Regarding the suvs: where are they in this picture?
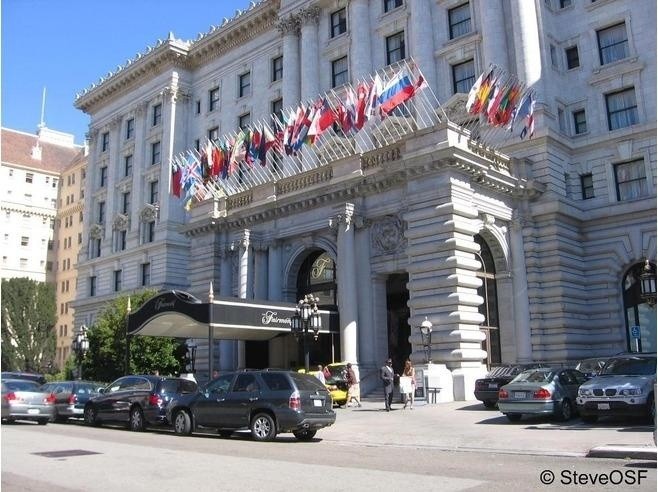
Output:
[164,367,337,443]
[1,371,45,385]
[575,351,656,426]
[40,381,98,424]
[82,374,199,433]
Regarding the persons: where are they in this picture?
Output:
[380,359,400,412]
[402,360,417,410]
[340,363,362,409]
[315,365,327,387]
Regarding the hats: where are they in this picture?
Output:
[384,358,393,364]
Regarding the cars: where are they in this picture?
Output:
[473,362,552,407]
[1,378,55,425]
[96,385,119,394]
[496,366,590,421]
[297,361,358,406]
[572,357,608,375]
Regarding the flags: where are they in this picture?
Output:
[171,59,428,198]
[466,71,537,140]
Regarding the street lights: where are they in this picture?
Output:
[640,260,656,307]
[71,324,91,381]
[288,292,322,373]
[416,316,435,362]
